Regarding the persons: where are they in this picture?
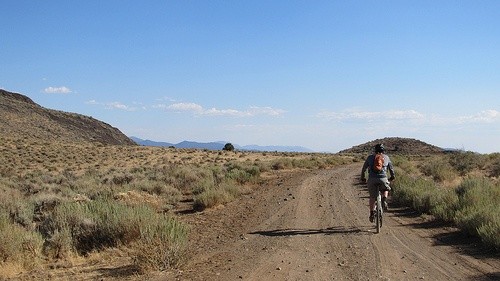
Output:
[359,143,395,224]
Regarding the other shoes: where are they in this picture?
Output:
[382,201,387,210]
[369,210,374,223]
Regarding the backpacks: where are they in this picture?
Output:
[368,154,387,173]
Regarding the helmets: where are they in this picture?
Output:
[375,144,385,151]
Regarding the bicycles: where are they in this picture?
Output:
[362,176,395,233]
